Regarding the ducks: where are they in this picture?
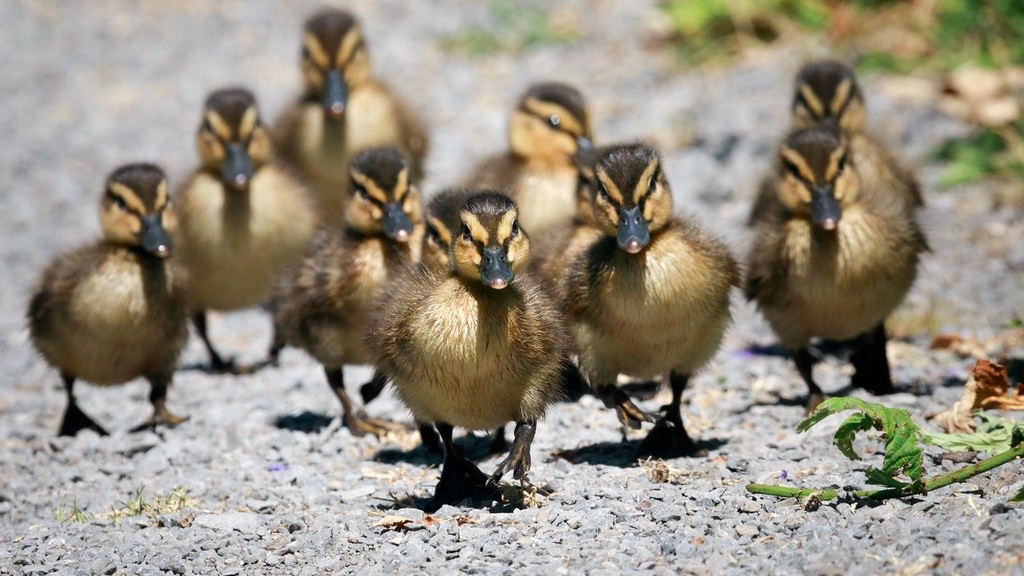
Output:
[25,162,192,434]
[538,139,750,457]
[271,7,429,182]
[273,141,427,437]
[466,79,595,275]
[741,62,931,416]
[364,187,570,511]
[162,88,324,373]
